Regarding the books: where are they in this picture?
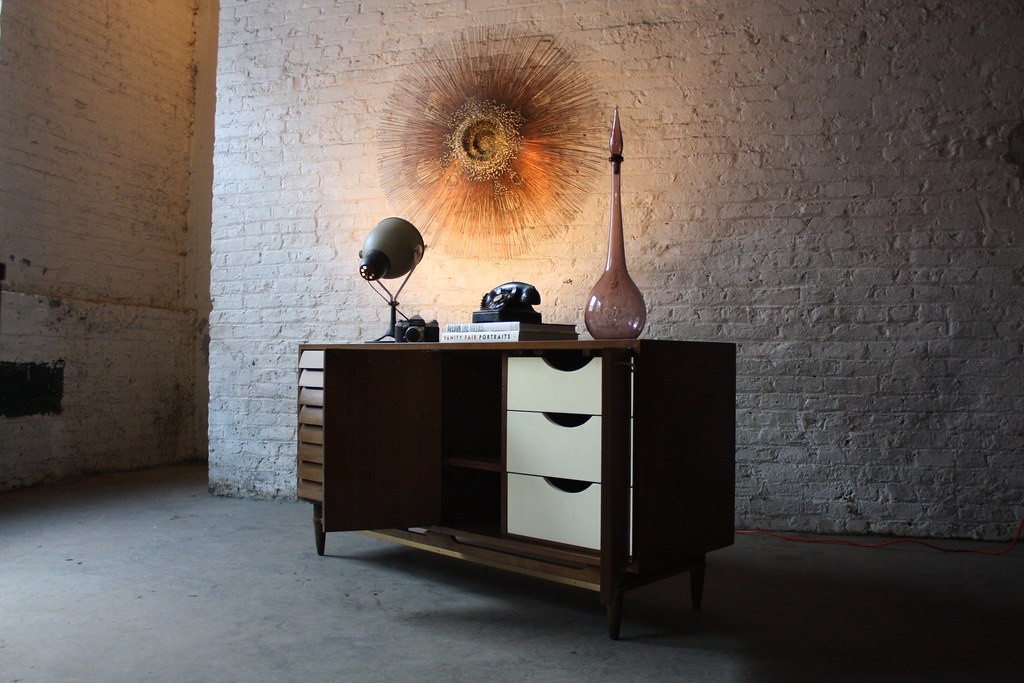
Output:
[438,321,581,343]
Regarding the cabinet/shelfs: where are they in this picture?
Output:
[293,339,738,640]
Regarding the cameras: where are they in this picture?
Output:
[394,314,439,342]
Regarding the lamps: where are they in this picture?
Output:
[359,216,424,343]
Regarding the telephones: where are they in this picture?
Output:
[472,282,542,324]
